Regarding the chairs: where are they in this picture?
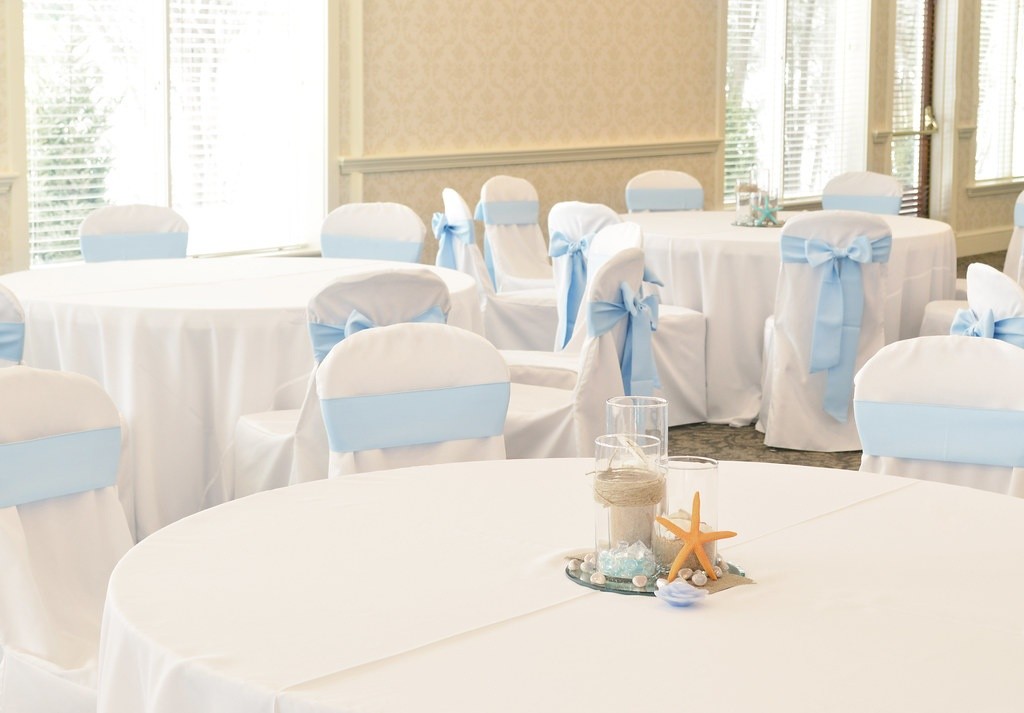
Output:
[0,169,1023,713]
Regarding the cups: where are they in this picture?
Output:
[650,456,718,575]
[750,168,769,217]
[593,433,661,583]
[737,178,761,223]
[768,187,778,218]
[606,396,669,518]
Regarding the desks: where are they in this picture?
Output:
[0,255,476,543]
[98,457,1022,713]
[619,208,957,429]
